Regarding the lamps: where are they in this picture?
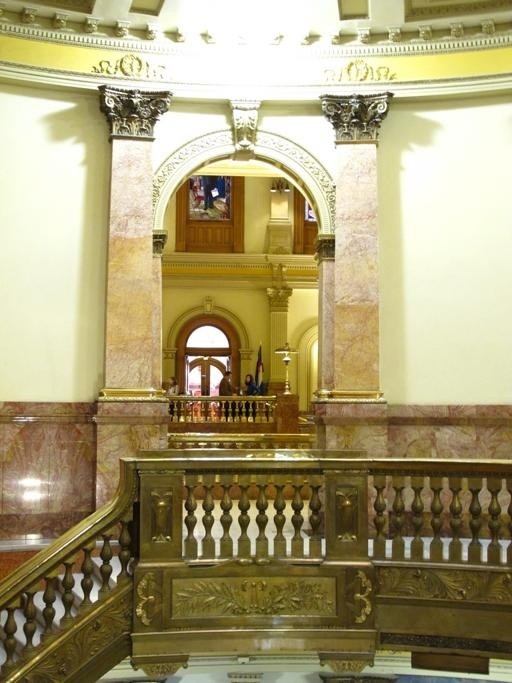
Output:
[269,177,290,194]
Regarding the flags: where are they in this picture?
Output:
[254,344,265,394]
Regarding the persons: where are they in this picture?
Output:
[190,175,230,213]
[244,373,261,395]
[166,376,181,396]
[219,370,239,396]
